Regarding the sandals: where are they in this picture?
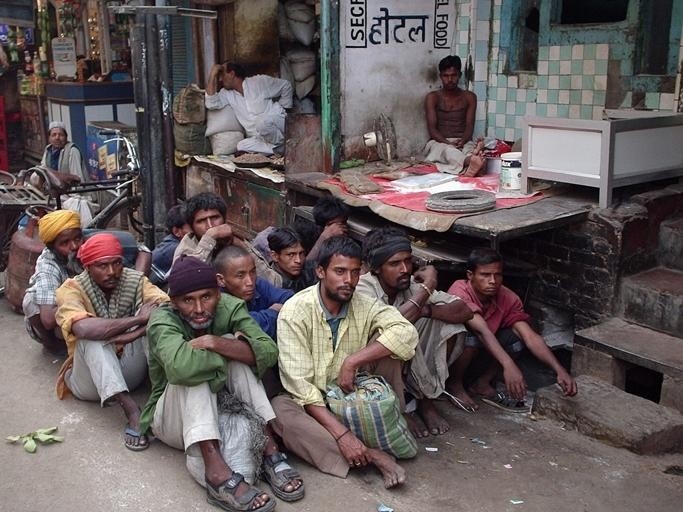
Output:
[259,452,304,502]
[204,470,276,512]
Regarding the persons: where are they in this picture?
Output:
[22,192,579,512]
[422,55,486,178]
[205,60,293,157]
[28,121,97,203]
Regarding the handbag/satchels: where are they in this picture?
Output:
[327,371,418,460]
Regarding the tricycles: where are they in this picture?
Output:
[1,128,144,275]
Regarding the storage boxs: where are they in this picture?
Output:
[87,121,135,197]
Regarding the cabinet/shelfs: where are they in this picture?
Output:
[18,96,49,168]
[45,81,135,179]
[184,160,287,242]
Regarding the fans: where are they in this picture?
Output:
[363,113,397,163]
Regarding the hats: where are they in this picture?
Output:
[49,120,67,131]
[168,254,217,298]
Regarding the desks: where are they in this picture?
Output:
[521,116,683,209]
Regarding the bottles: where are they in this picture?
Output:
[0,2,80,98]
[106,14,130,72]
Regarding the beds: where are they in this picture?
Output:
[318,165,570,251]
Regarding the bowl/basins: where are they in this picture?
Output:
[232,158,284,171]
[485,158,502,174]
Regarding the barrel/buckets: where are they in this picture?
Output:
[500,151,522,191]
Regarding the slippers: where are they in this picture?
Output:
[481,392,530,413]
[124,422,149,451]
